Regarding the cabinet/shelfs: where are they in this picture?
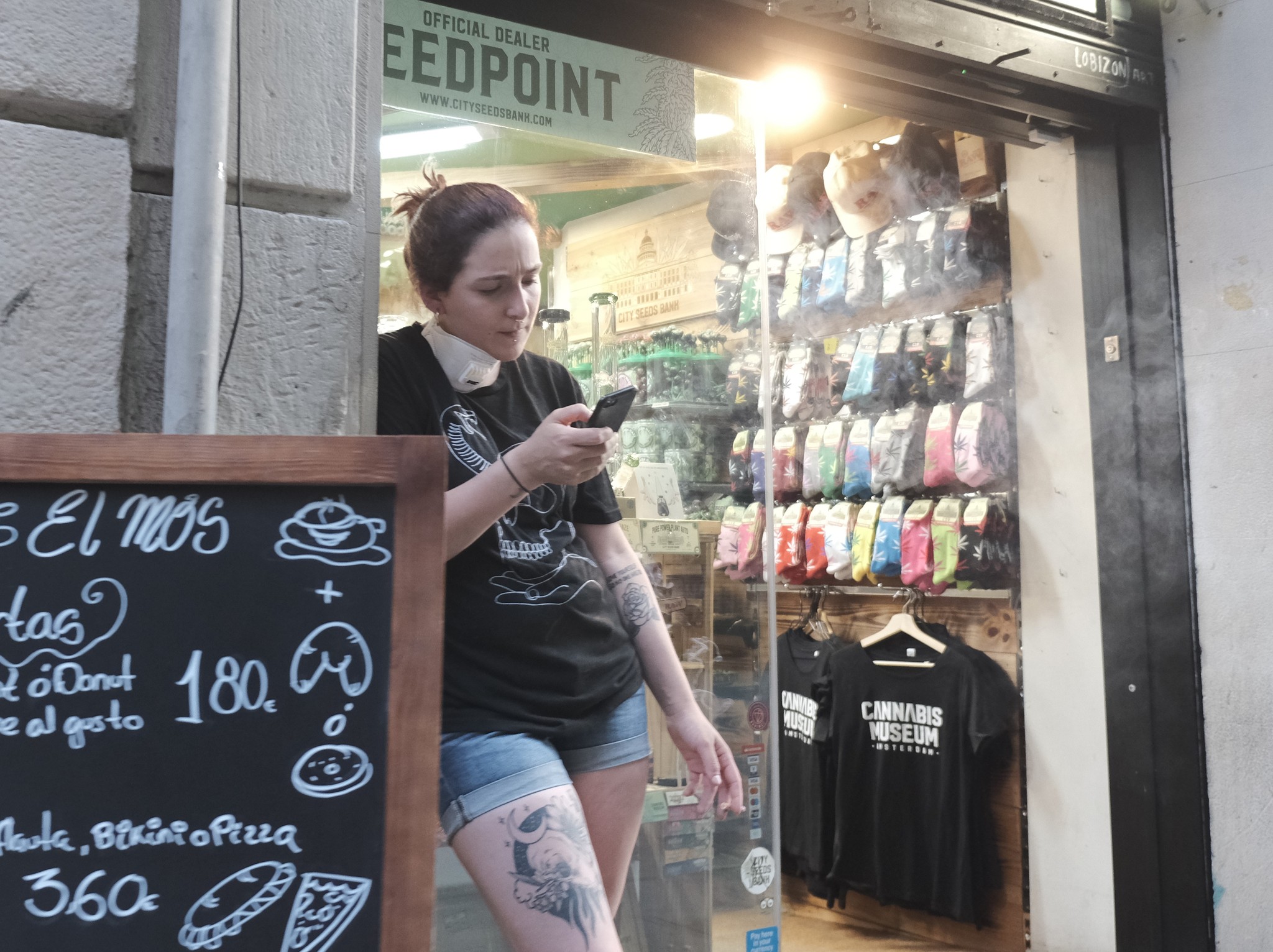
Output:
[565,347,734,515]
[620,519,770,904]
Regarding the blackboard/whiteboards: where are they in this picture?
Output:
[0,433,448,952]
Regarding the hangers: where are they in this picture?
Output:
[788,587,948,668]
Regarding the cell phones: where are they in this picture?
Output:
[585,385,637,432]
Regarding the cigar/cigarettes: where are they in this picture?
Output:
[721,804,744,813]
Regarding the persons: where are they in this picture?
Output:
[376,167,745,952]
